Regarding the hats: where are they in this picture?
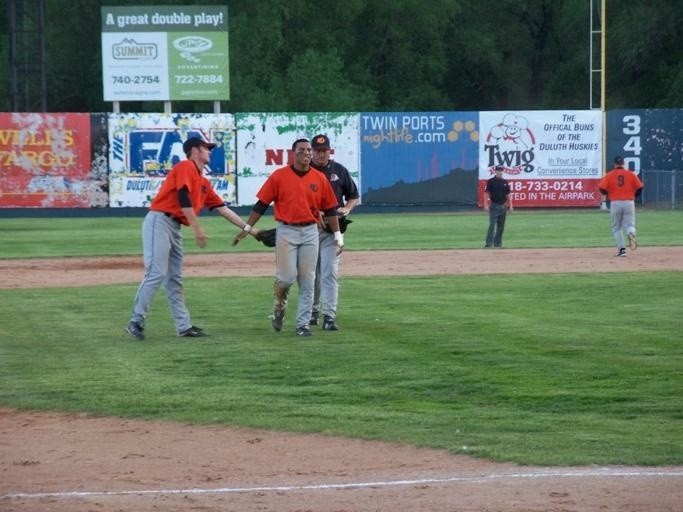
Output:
[183,137,217,153]
[311,135,330,151]
[613,156,624,165]
[495,165,504,170]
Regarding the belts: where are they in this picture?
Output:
[278,219,315,227]
[164,212,182,224]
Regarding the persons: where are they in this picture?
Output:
[232,138,344,336]
[598,155,644,257]
[482,165,513,249]
[307,133,361,331]
[123,138,275,343]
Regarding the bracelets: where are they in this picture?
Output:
[243,223,251,232]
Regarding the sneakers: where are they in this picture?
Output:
[295,324,314,337]
[177,325,212,337]
[323,316,340,332]
[124,321,144,341]
[616,249,627,257]
[485,244,501,248]
[309,312,320,326]
[628,232,637,251]
[272,310,284,332]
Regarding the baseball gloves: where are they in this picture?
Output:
[256,228,276,247]
